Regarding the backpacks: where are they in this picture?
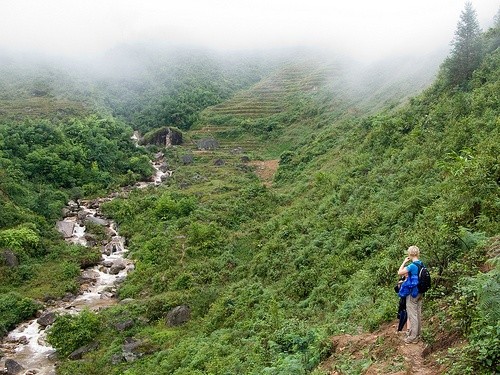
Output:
[408,262,431,293]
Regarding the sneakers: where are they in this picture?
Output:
[404,337,417,344]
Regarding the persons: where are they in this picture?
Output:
[394,265,410,332]
[395,245,428,345]
